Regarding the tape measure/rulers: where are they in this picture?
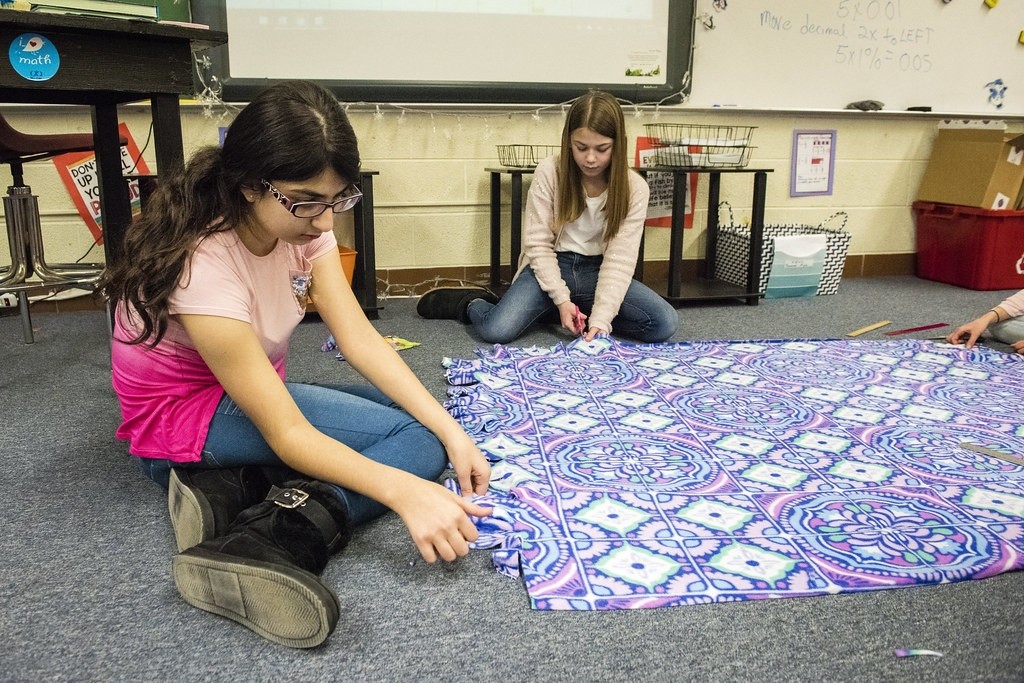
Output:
[960,442,1024,467]
[845,320,892,337]
[883,323,950,335]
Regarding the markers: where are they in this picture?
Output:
[712,104,737,107]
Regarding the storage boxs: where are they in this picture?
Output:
[916,119,1024,210]
[912,201,1024,291]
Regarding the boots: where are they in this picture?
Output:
[172,481,350,648]
[168,465,304,553]
[417,287,499,324]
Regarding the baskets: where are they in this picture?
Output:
[714,201,852,295]
[646,121,759,170]
[497,145,562,168]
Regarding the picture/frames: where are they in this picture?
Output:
[789,129,837,197]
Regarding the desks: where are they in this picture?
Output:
[123,168,383,319]
[484,164,775,311]
[0,11,229,334]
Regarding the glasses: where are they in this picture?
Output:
[258,178,363,218]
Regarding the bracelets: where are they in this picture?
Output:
[990,309,1000,324]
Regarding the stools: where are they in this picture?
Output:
[0,105,128,345]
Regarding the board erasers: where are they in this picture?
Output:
[907,107,931,111]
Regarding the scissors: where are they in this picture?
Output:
[575,306,584,340]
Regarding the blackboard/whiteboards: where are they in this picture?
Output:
[682,0,1024,122]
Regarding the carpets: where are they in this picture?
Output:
[439,335,1024,616]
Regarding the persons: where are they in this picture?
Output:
[945,289,1024,354]
[416,90,679,345]
[90,79,493,649]
